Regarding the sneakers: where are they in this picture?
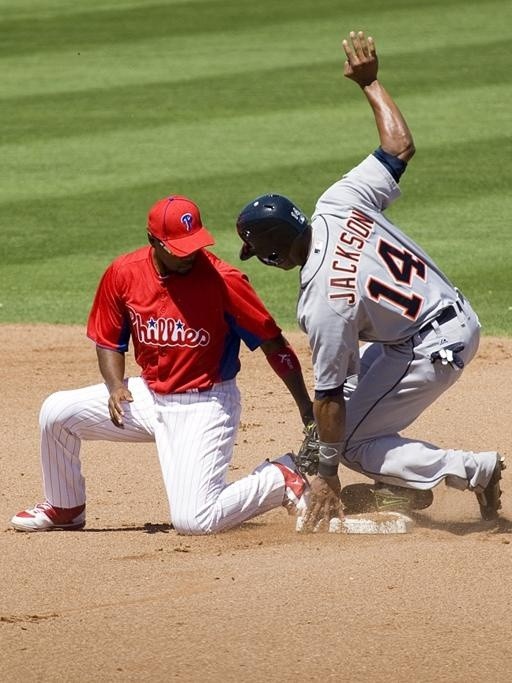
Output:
[341,484,433,513]
[11,502,86,532]
[474,452,507,520]
[270,453,313,532]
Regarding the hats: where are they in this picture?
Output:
[148,195,214,258]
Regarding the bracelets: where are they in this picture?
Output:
[303,406,315,426]
[313,437,345,479]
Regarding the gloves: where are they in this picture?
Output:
[431,345,464,371]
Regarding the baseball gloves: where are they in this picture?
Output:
[294,408,319,477]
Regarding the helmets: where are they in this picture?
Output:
[236,195,309,266]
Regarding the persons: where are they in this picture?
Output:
[10,194,316,535]
[234,29,506,535]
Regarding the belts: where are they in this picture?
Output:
[419,288,463,333]
[175,384,213,393]
